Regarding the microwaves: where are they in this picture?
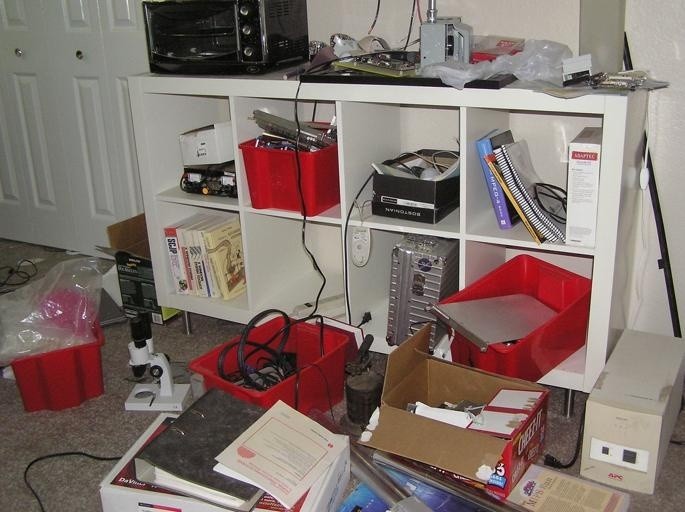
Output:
[142,1,310,76]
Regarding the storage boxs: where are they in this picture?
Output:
[236,118,340,216]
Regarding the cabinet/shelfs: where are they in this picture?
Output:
[129,70,647,417]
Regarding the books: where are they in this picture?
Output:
[475,129,566,246]
[133,387,631,512]
[163,212,247,301]
